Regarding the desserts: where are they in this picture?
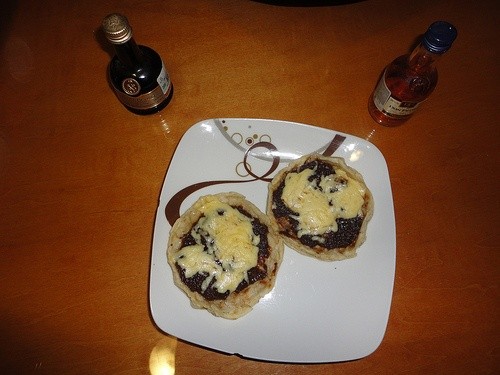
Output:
[166,191,285,320]
[267,152,374,261]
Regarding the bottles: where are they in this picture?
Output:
[103,12,174,117]
[367,20,460,128]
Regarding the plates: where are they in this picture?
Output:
[149,117,398,363]
